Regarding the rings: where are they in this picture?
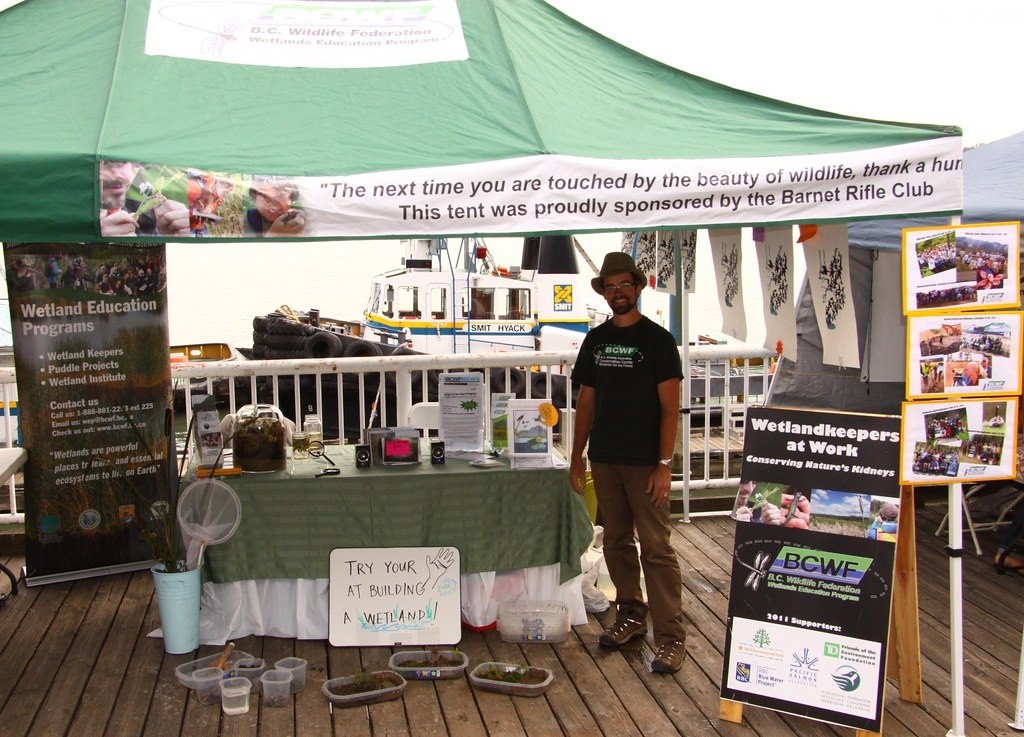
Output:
[664,494,668,497]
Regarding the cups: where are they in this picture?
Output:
[219,676,252,716]
[274,656,308,693]
[191,666,224,706]
[260,669,294,706]
[233,657,266,693]
[151,559,202,654]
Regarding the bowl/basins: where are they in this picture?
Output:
[175,649,254,689]
[497,599,572,644]
[470,661,554,697]
[388,650,469,681]
[320,669,407,709]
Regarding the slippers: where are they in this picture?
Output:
[992,557,1024,576]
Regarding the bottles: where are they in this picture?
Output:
[292,432,310,459]
[304,414,323,450]
[232,404,288,474]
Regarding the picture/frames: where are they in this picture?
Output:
[906,311,1024,398]
[901,221,1020,316]
[899,397,1019,486]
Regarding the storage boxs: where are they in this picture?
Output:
[586,542,648,603]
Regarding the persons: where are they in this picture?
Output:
[781,493,810,529]
[917,242,956,274]
[867,502,898,541]
[188,171,235,236]
[6,250,166,295]
[569,251,687,673]
[99,159,191,237]
[916,285,977,305]
[242,176,308,236]
[913,414,1004,476]
[959,248,1006,290]
[919,333,1003,388]
[736,479,780,525]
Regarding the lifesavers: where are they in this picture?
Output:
[386,347,421,385]
[303,332,342,358]
[494,367,526,394]
[264,316,313,334]
[492,265,523,279]
[264,346,314,392]
[531,372,559,398]
[429,369,439,385]
[344,338,385,384]
[262,333,308,350]
[251,314,268,359]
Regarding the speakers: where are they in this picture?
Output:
[431,442,445,464]
[356,445,371,468]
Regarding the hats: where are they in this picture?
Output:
[591,252,647,295]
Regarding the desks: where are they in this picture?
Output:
[182,445,595,645]
[0,448,28,595]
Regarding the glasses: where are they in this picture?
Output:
[603,281,636,293]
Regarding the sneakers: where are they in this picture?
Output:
[650,638,686,673]
[598,609,648,646]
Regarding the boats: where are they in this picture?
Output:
[243,234,601,413]
[169,342,240,411]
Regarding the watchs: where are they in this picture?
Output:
[658,459,674,469]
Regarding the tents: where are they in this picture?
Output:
[764,130,1024,415]
[0,0,963,737]
